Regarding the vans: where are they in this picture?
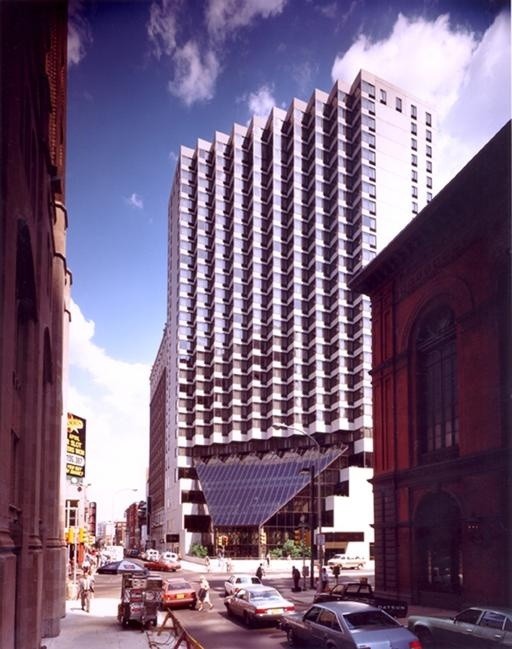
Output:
[327,554,366,570]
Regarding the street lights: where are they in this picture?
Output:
[273,423,322,591]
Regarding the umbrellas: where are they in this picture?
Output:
[98,559,149,574]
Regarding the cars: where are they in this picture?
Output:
[224,574,512,649]
[159,577,197,611]
[124,549,181,572]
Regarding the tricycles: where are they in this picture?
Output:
[118,573,162,632]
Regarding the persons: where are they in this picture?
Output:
[256,563,266,579]
[205,556,211,573]
[266,553,270,564]
[197,576,214,610]
[226,557,233,571]
[291,566,301,586]
[322,567,328,589]
[76,569,96,613]
[82,559,90,571]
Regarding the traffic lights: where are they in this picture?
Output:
[67,526,87,545]
